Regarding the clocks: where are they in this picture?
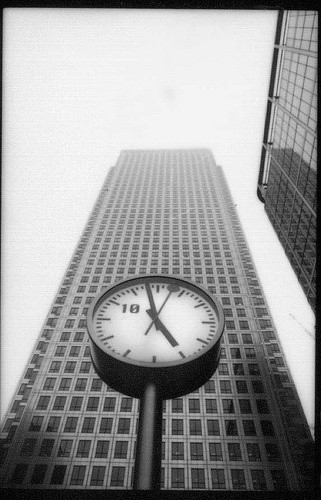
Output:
[86,272,227,369]
